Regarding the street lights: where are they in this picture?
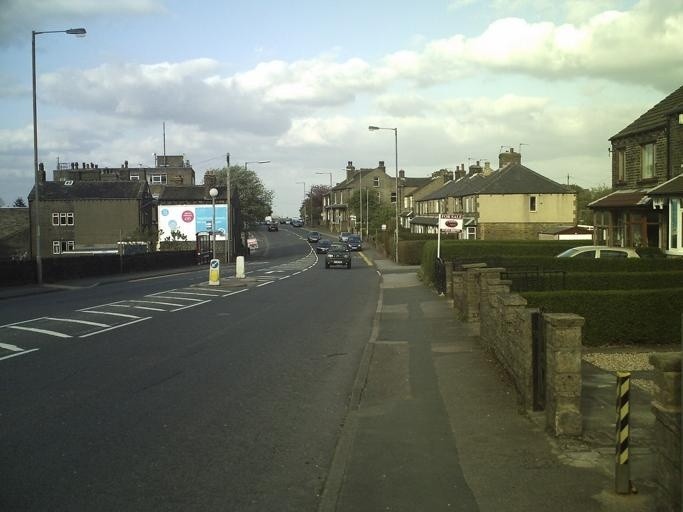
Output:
[368,125,399,266]
[294,181,305,216]
[315,172,332,231]
[245,160,271,170]
[209,188,218,259]
[32,28,87,284]
[340,168,362,231]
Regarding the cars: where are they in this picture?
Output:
[306,231,361,269]
[264,216,304,232]
[537,225,607,241]
[555,246,640,258]
